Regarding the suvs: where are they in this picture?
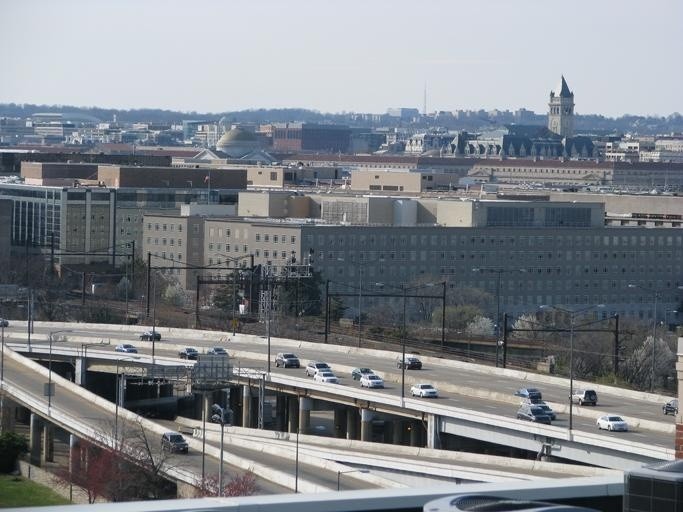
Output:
[568,390,597,406]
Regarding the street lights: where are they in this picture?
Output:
[115,356,141,437]
[628,283,682,393]
[49,329,73,405]
[202,388,230,496]
[238,269,297,372]
[296,426,325,494]
[1,304,24,381]
[471,267,526,367]
[539,303,605,432]
[337,257,387,348]
[337,469,369,491]
[375,281,434,399]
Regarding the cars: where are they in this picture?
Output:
[139,331,160,341]
[114,344,136,353]
[0,318,8,327]
[178,347,198,359]
[160,433,188,453]
[275,353,438,399]
[662,400,677,416]
[596,416,628,432]
[207,347,227,356]
[517,387,555,425]
